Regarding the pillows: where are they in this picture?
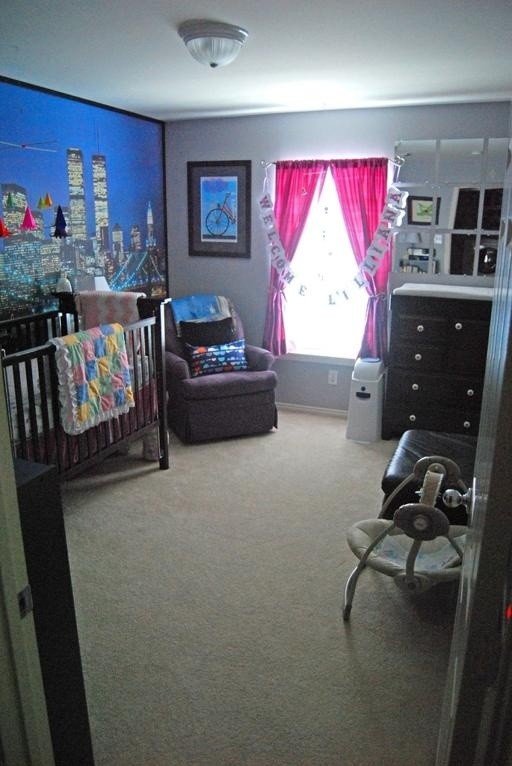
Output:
[185,337,249,378]
[179,316,236,349]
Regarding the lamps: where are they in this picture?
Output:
[180,23,249,71]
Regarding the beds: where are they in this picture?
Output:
[0,291,172,484]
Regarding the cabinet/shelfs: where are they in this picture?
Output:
[381,294,493,441]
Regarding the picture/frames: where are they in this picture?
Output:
[186,159,252,258]
[407,195,441,226]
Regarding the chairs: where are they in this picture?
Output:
[158,295,279,445]
[375,428,477,524]
